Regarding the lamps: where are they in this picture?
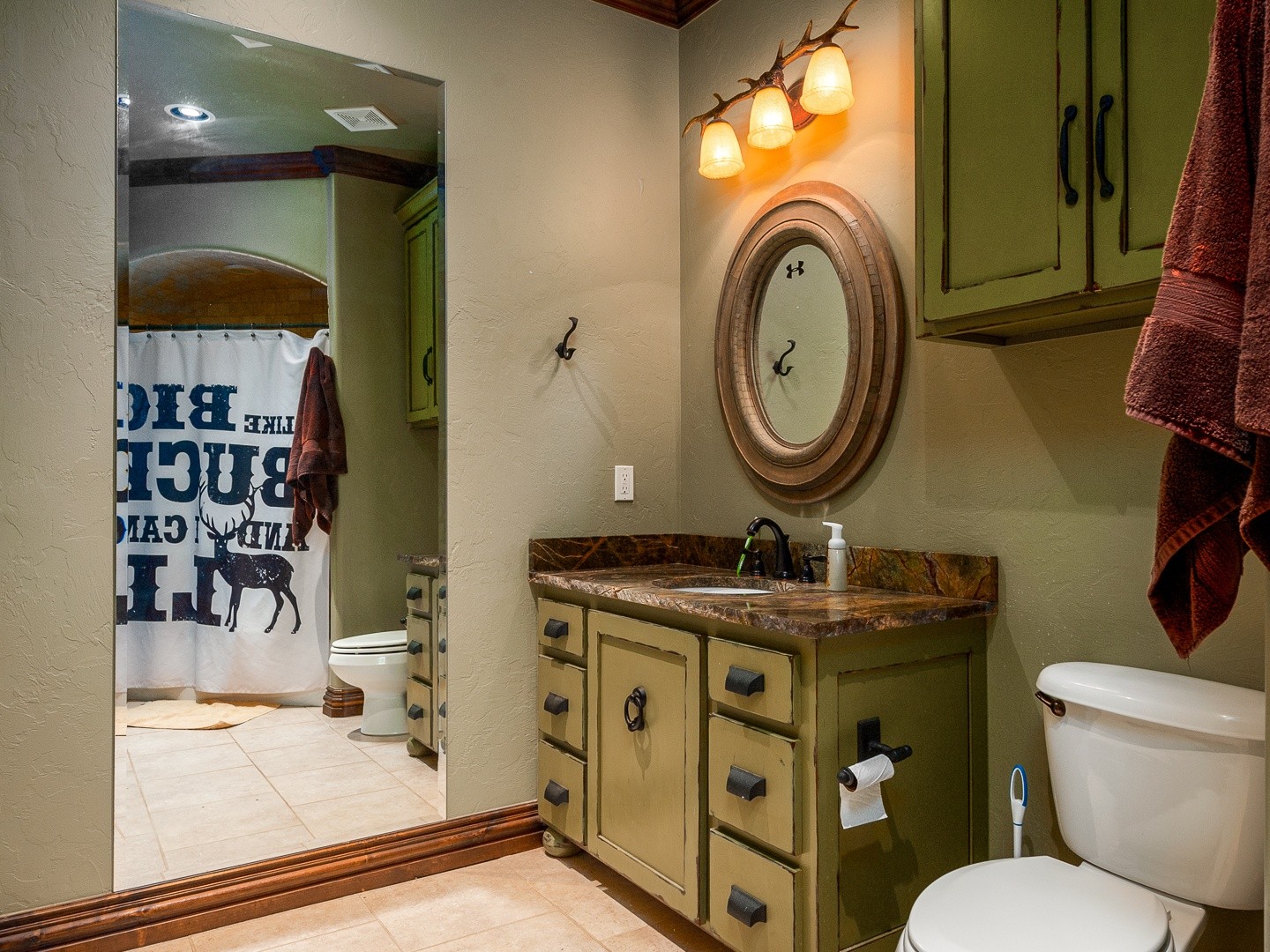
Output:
[698,117,745,179]
[747,83,797,150]
[800,41,854,115]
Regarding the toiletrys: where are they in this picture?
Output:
[821,520,852,593]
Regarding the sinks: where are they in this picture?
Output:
[652,574,798,597]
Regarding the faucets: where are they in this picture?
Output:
[747,517,797,580]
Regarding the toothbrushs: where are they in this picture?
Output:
[736,515,762,576]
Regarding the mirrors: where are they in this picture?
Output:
[713,179,906,503]
[116,0,449,892]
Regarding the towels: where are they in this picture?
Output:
[1119,2,1269,659]
[285,346,349,549]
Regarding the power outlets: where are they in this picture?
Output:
[615,466,634,500]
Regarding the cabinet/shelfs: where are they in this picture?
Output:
[529,531,998,951]
[914,0,1216,351]
[396,554,445,758]
[393,176,440,429]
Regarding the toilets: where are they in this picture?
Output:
[889,661,1270,952]
[327,623,420,739]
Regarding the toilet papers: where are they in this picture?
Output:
[836,754,897,831]
